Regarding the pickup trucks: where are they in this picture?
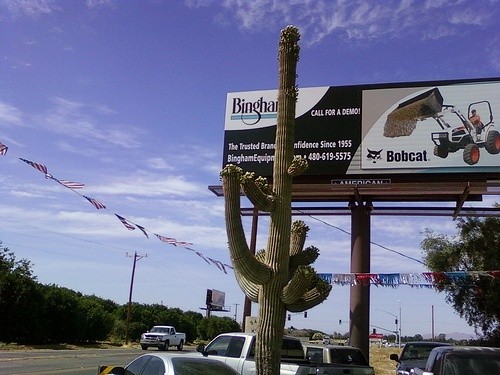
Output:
[139,325,186,350]
[185,332,376,375]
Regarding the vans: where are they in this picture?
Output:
[302,344,369,366]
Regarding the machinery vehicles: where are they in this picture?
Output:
[386,86,500,166]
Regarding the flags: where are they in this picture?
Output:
[0,142,8,155]
[83,195,107,209]
[19,157,53,179]
[137,224,194,246]
[58,180,85,189]
[186,247,228,275]
[115,213,135,230]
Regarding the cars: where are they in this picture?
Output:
[323,337,330,344]
[407,345,500,375]
[390,341,452,375]
[97,352,241,375]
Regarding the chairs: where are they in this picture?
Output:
[226,337,241,356]
[410,350,418,358]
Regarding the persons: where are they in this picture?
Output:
[469,109,480,124]
